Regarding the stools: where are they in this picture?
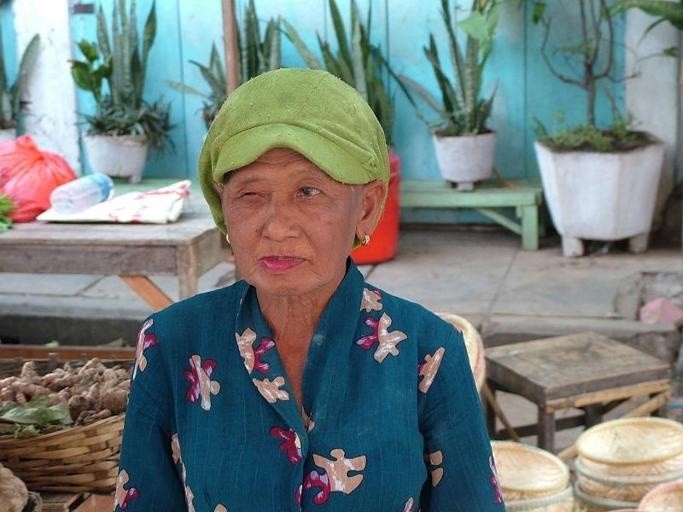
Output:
[398,179,546,248]
[478,329,673,479]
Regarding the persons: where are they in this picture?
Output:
[112,67,506,512]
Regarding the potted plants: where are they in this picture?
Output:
[532,0,679,260]
[396,0,508,193]
[253,0,396,258]
[2,0,40,143]
[66,0,181,189]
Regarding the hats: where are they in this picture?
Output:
[197,67,392,254]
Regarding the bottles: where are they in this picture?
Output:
[48,173,114,214]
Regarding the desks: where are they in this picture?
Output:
[0,201,232,362]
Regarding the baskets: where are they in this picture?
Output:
[488,416,683,512]
[429,309,488,397]
[0,352,139,497]
